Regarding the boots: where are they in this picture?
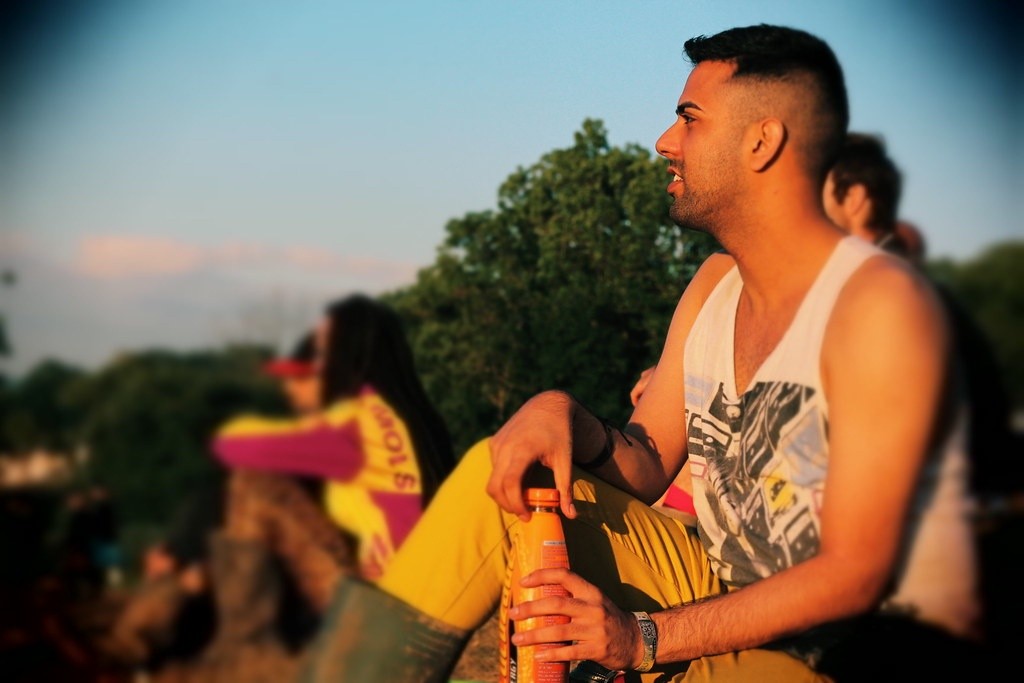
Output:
[293,576,474,683]
[189,533,289,683]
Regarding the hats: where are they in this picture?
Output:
[264,317,328,379]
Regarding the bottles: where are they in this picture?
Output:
[513,487,571,682]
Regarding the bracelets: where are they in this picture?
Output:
[580,417,615,470]
[635,610,657,675]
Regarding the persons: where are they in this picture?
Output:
[296,22,984,683]
[0,294,456,682]
